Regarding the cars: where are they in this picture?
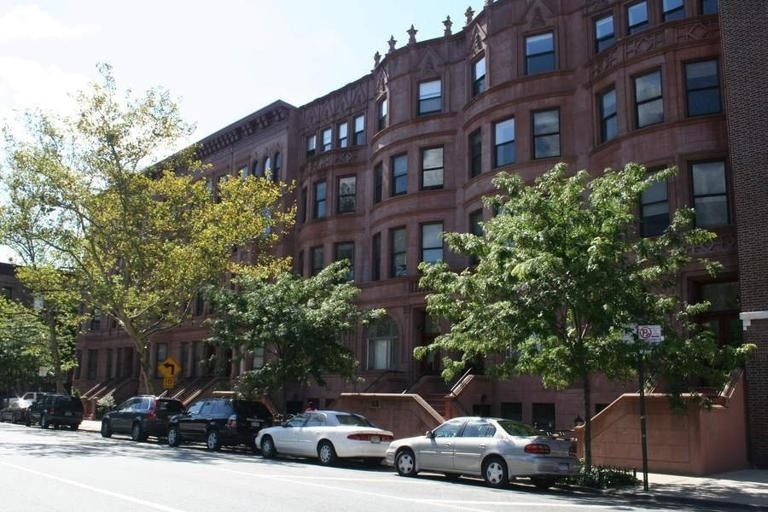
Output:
[383,416,577,488]
[255,410,393,465]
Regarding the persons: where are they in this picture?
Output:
[304,402,318,413]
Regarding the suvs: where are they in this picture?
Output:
[166,397,273,451]
[11,392,48,408]
[101,395,184,440]
[25,394,84,430]
